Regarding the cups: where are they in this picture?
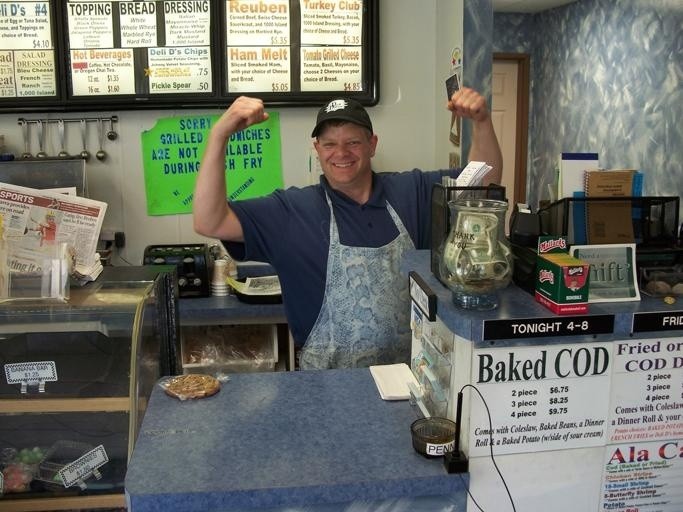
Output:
[211,242,238,297]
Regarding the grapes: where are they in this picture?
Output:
[13,446,43,464]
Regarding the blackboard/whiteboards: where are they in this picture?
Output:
[0,0,379,113]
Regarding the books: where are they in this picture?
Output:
[441,160,493,204]
[9,252,103,291]
[537,151,644,246]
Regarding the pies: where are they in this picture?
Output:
[166,374,220,400]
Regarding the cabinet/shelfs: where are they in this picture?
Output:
[2,266,171,511]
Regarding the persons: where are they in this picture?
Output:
[189,85,504,371]
[27,211,56,248]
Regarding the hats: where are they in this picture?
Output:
[311,98,372,139]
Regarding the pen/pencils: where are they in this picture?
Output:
[454,393,462,455]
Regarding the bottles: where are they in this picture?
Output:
[439,197,515,312]
